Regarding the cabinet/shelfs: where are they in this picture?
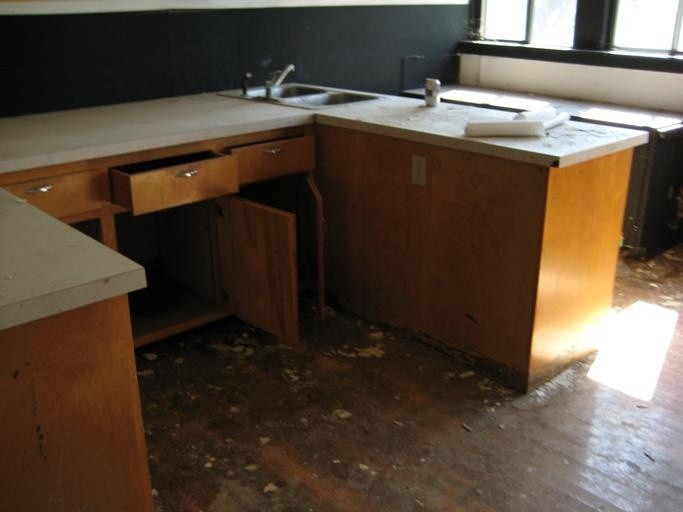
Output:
[108,132,330,346]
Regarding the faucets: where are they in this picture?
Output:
[266,64,295,98]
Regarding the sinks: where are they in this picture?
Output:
[278,91,379,109]
[216,83,326,98]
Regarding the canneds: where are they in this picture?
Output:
[425,79,441,107]
